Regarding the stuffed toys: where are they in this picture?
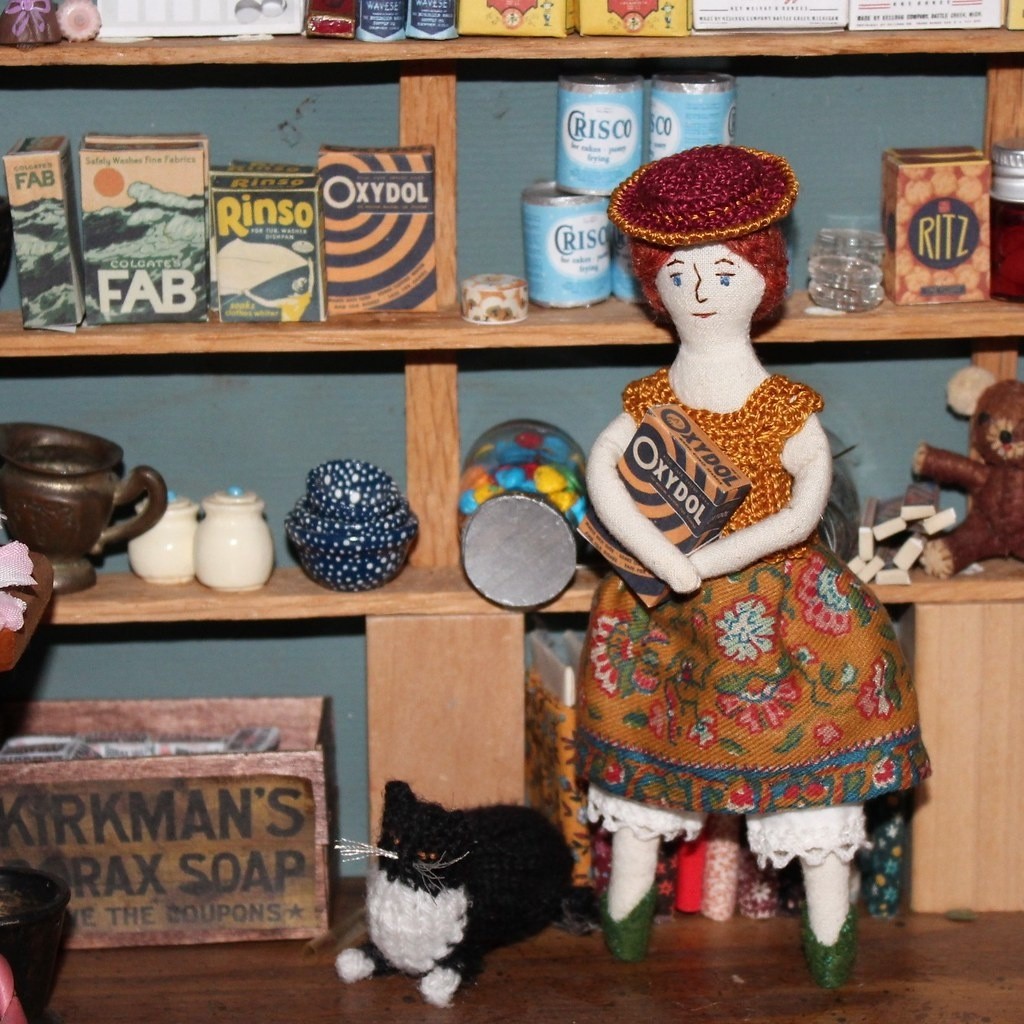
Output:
[334,780,574,1007]
[914,364,1024,578]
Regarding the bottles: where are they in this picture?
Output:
[990,139,1024,306]
[285,461,420,592]
[194,486,274,592]
[457,418,588,608]
[127,490,199,585]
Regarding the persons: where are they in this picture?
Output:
[575,144,934,987]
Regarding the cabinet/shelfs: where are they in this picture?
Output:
[0,26,1023,915]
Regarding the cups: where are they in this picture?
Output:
[0,422,168,594]
[806,227,886,309]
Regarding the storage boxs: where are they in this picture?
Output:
[1,137,84,328]
[883,146,992,305]
[82,133,210,231]
[206,161,320,324]
[78,142,210,322]
[0,695,337,945]
[316,144,439,316]
